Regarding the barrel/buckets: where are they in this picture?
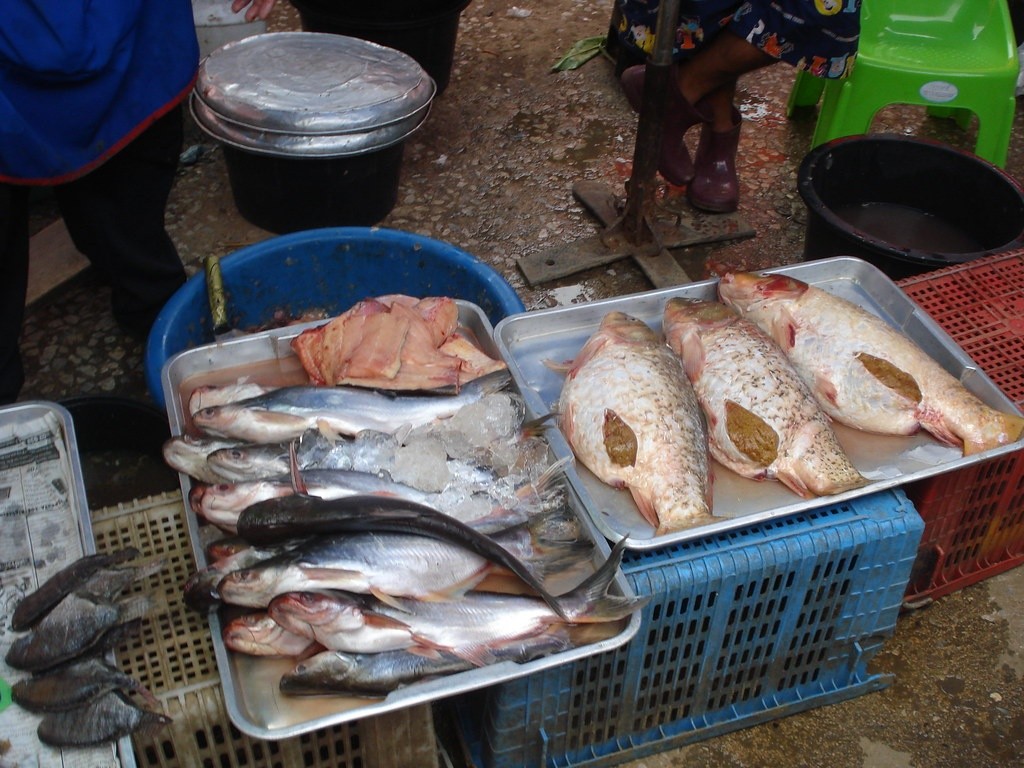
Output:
[227,137,402,234]
[800,132,1024,280]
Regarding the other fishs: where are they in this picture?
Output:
[4,546,175,747]
[160,370,653,701]
[553,267,1024,540]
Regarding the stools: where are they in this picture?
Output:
[785,0,1020,172]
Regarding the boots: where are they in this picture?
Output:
[621,63,742,212]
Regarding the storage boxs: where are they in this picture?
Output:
[437,487,926,768]
[888,250,1024,608]
[91,490,437,768]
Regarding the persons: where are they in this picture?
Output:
[0,1,278,414]
[623,1,862,216]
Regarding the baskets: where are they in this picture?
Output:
[90,491,440,767]
[894,246,1024,612]
[456,490,923,768]
